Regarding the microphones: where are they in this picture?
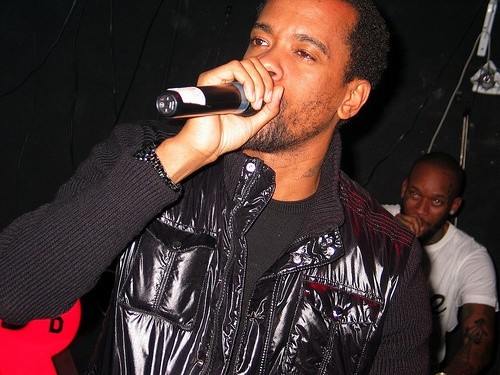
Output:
[156,82,267,118]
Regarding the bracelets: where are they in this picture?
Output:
[133,141,184,198]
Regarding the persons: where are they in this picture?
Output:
[381,150,497,375]
[0,0,435,375]
